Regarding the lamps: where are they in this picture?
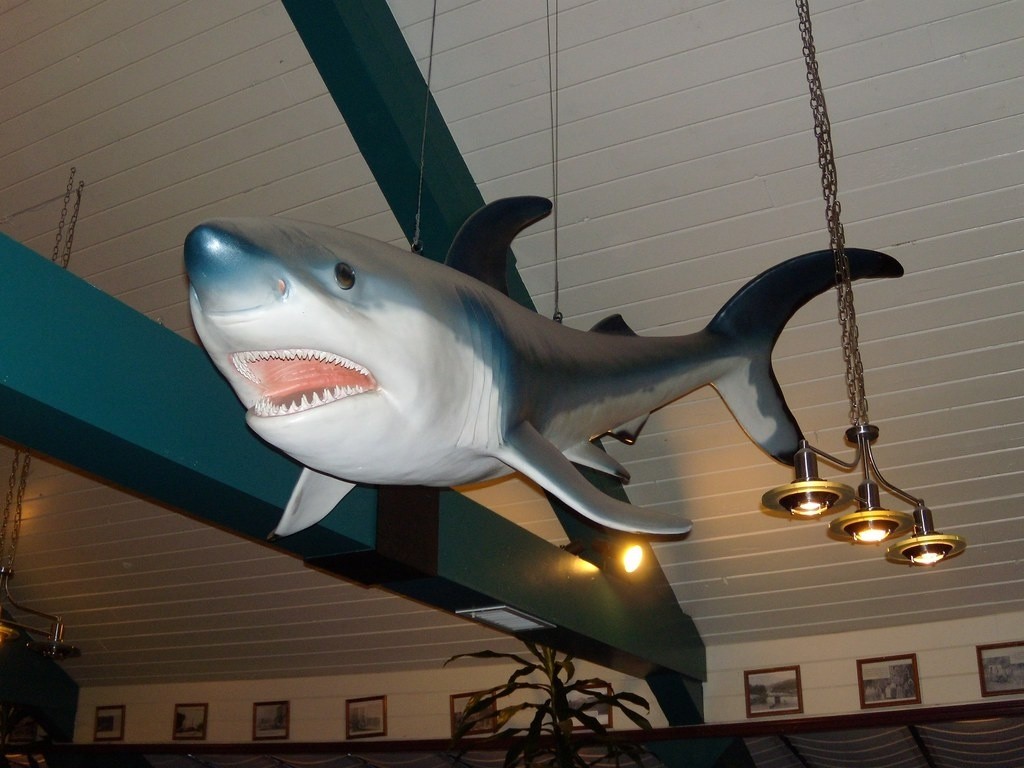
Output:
[0,166,84,660]
[762,0,968,565]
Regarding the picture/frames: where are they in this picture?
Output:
[449,690,498,739]
[345,695,388,740]
[555,683,613,730]
[252,701,290,741]
[976,640,1024,697]
[856,652,922,710]
[92,705,126,742]
[172,702,209,741]
[744,665,804,718]
